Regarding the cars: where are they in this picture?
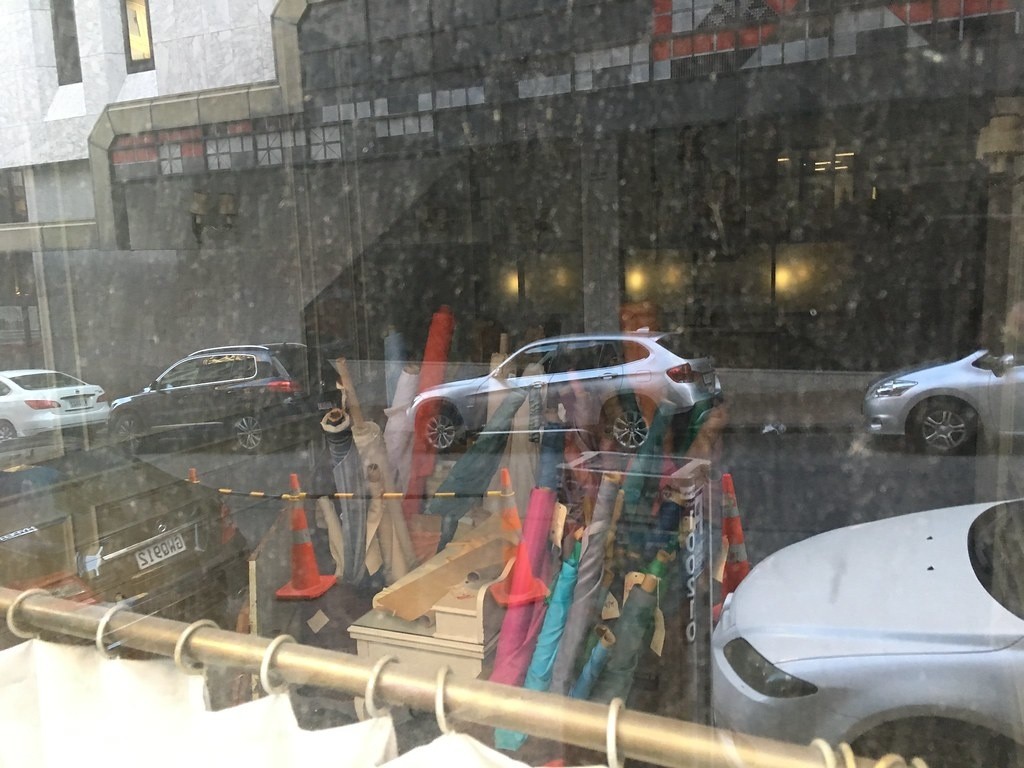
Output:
[708,499,1022,759]
[0,370,110,438]
[861,327,1022,452]
[1,435,254,630]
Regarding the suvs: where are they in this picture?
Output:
[113,343,347,453]
[408,326,726,458]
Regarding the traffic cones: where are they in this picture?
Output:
[495,469,550,606]
[720,473,748,586]
[276,473,334,601]
